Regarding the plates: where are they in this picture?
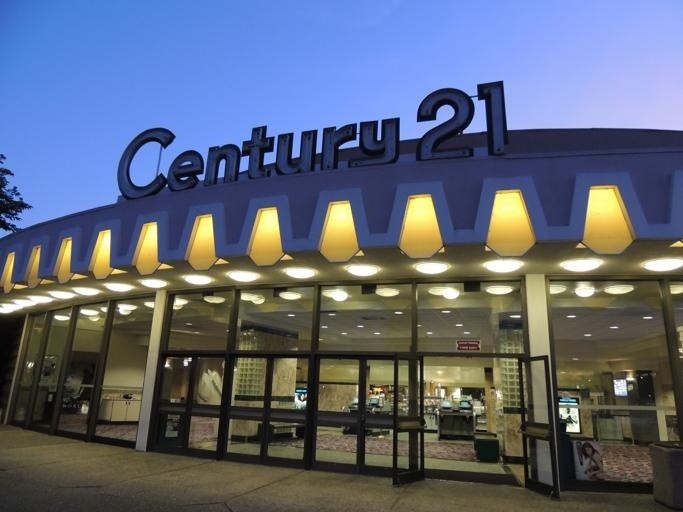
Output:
[97,397,142,422]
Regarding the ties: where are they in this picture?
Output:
[369,398,379,404]
[440,400,452,409]
[352,398,359,404]
[459,401,472,409]
[612,379,628,397]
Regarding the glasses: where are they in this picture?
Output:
[49,290,75,300]
[481,258,524,273]
[72,286,103,298]
[560,257,604,272]
[411,260,451,274]
[640,257,683,273]
[183,273,215,286]
[104,282,134,293]
[226,269,259,283]
[283,265,317,279]
[344,262,381,278]
[138,279,169,289]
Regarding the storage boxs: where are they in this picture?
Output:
[471,431,499,463]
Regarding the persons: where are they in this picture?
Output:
[297,393,307,409]
[581,442,602,481]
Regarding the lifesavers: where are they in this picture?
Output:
[460,409,473,413]
[441,409,453,412]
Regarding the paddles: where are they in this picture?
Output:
[290,433,500,464]
